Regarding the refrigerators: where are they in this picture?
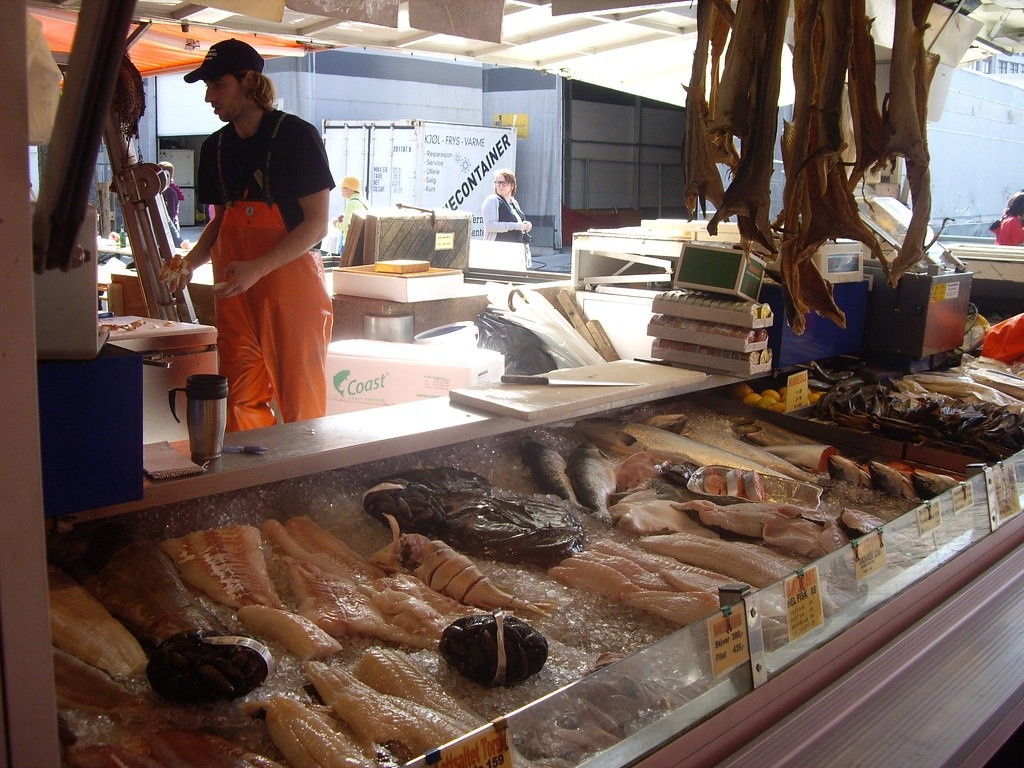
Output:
[100,314,220,445]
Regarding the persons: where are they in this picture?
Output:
[159,36,338,430]
[482,170,533,243]
[157,160,183,236]
[993,191,1024,245]
[333,176,371,257]
[151,169,183,248]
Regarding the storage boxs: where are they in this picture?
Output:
[648,324,768,353]
[651,347,772,375]
[675,244,766,303]
[324,339,505,416]
[652,300,773,329]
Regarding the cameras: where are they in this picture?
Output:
[516,233,534,244]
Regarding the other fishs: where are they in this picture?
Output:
[575,416,797,485]
[520,436,592,516]
[564,438,618,525]
[728,416,960,503]
[902,366,1024,407]
[639,414,688,435]
[685,429,831,494]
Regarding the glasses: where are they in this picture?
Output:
[494,180,510,186]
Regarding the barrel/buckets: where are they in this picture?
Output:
[413,321,480,348]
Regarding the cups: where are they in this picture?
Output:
[167,374,230,461]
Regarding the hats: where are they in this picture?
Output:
[182,37,265,86]
[337,175,361,193]
